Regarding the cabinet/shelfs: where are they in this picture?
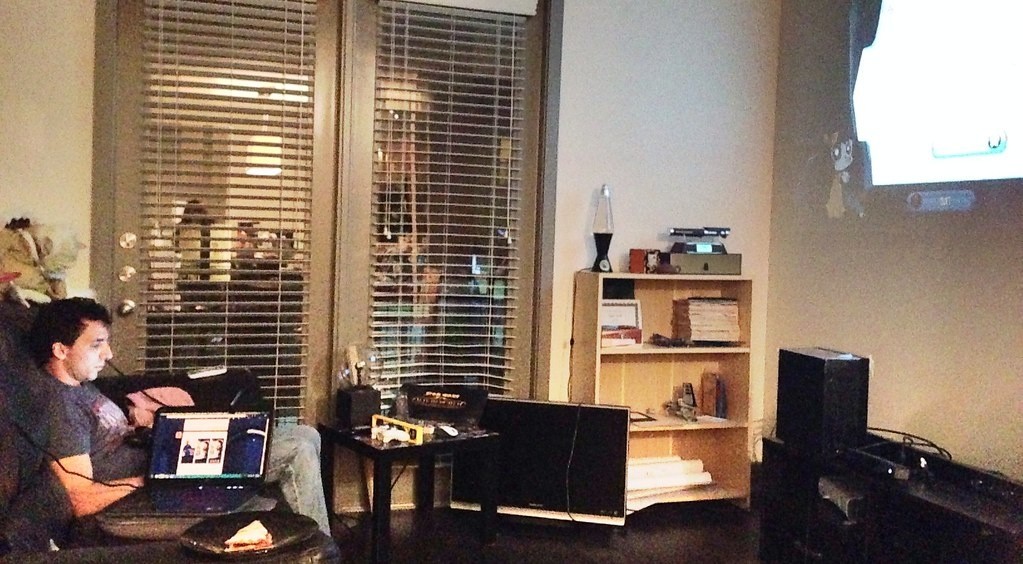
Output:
[569,270,755,512]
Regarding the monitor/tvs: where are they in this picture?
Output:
[450,398,630,526]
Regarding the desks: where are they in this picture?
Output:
[317,421,500,564]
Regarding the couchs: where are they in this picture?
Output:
[0,299,338,564]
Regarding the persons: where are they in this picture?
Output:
[28,297,332,547]
[183,440,192,456]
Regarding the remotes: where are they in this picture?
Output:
[188,366,227,379]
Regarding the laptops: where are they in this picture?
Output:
[102,405,277,517]
